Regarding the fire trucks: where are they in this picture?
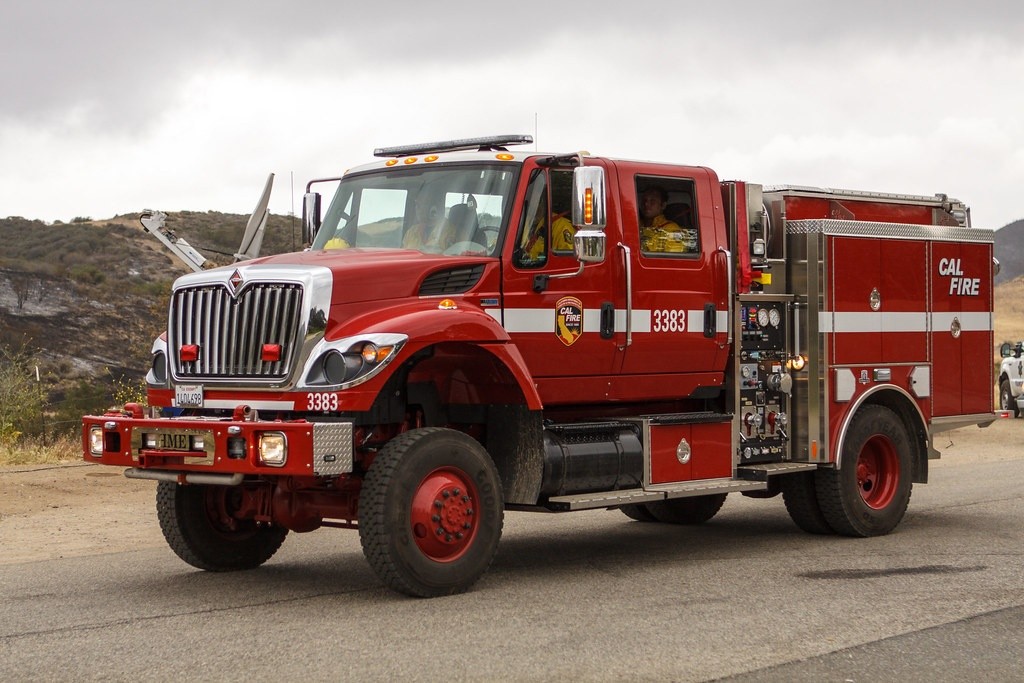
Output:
[81,134,1013,598]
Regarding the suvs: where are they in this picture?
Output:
[999,340,1024,416]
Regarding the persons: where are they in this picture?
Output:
[639,184,689,253]
[521,195,576,263]
[402,191,457,254]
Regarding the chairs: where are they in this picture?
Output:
[664,203,693,229]
[551,194,572,220]
[448,203,478,251]
